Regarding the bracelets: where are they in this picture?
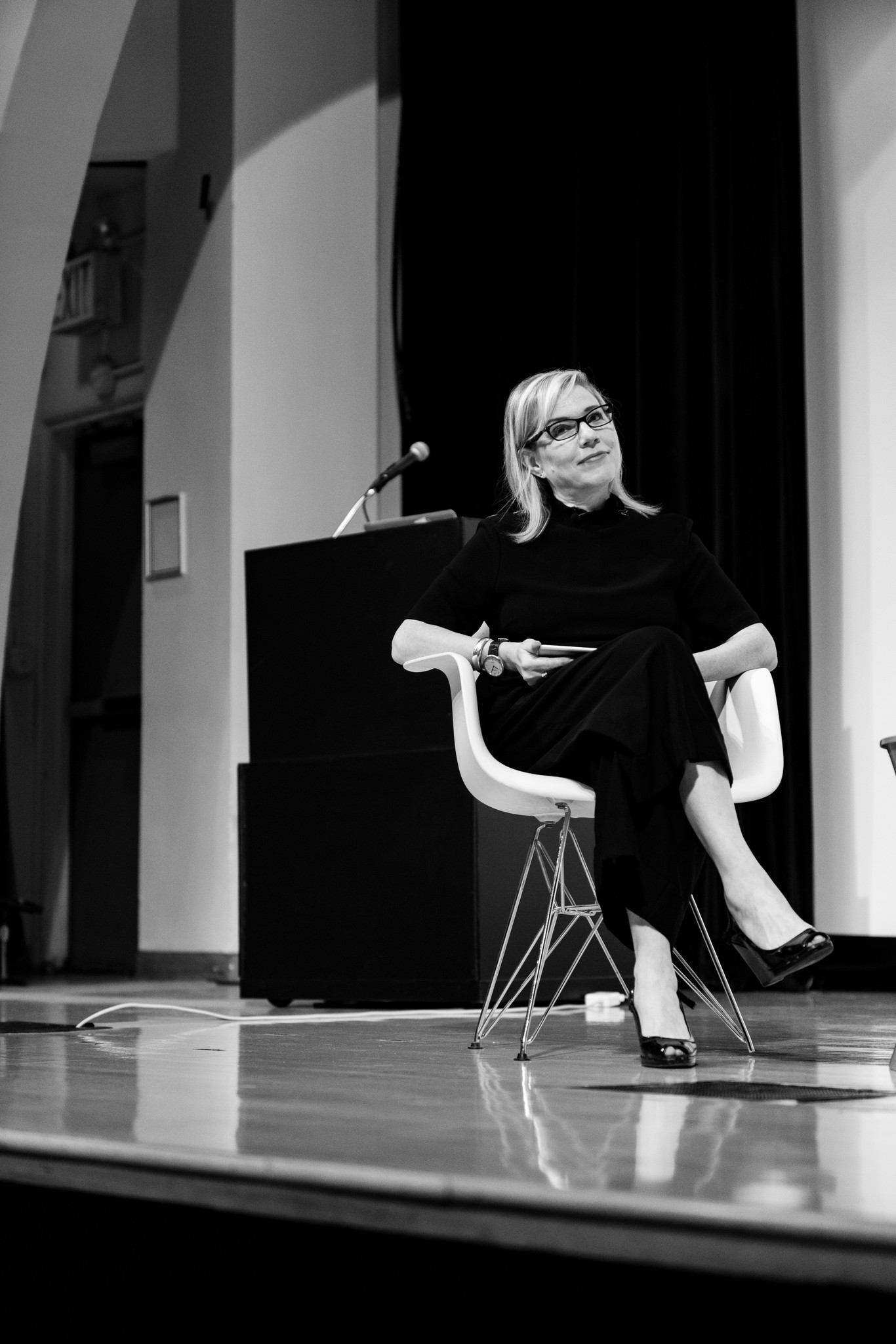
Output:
[472,638,493,673]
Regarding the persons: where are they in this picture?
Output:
[390,368,835,1068]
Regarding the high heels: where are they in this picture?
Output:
[629,985,697,1068]
[725,911,834,985]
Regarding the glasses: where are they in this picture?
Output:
[526,402,615,450]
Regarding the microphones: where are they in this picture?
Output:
[370,442,431,489]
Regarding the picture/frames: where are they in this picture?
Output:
[143,491,189,579]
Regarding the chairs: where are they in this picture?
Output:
[401,651,784,1063]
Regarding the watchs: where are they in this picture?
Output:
[483,638,511,677]
